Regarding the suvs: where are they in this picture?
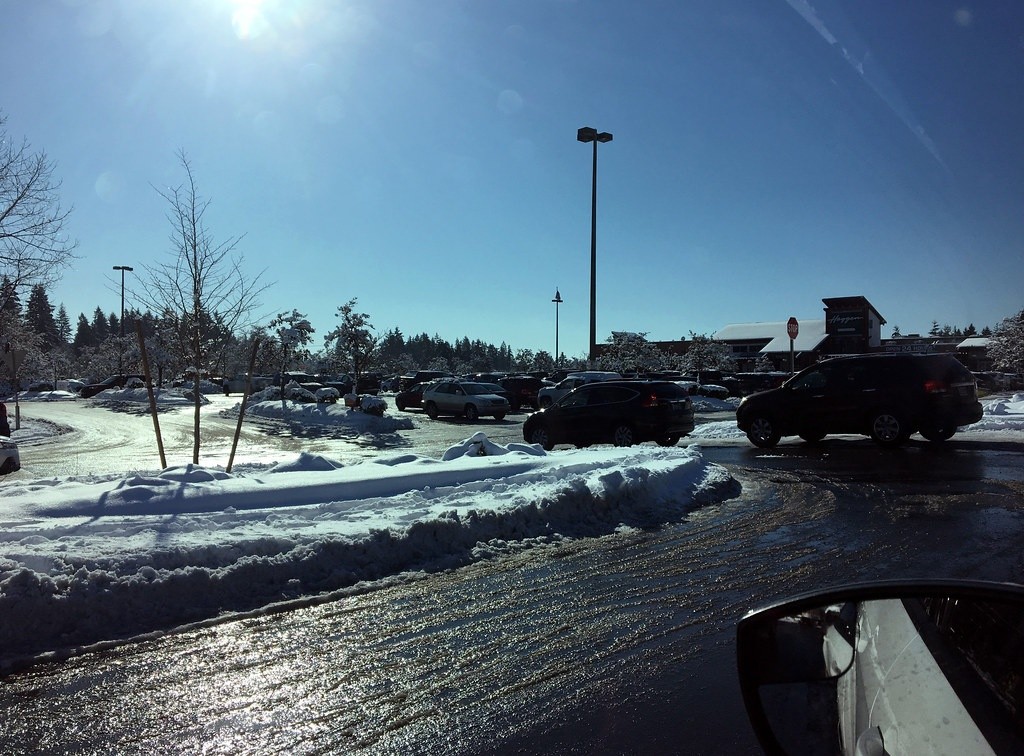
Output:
[81,373,153,399]
[735,351,985,449]
[422,379,511,424]
[523,380,695,451]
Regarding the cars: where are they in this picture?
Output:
[395,381,430,413]
[223,369,623,406]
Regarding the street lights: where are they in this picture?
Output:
[112,266,134,379]
[577,127,614,368]
[552,287,563,365]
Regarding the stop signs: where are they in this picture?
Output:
[787,317,798,339]
